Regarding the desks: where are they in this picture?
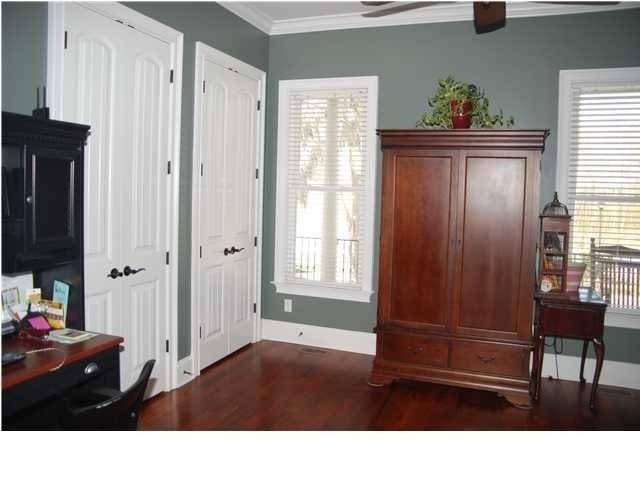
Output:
[2,328,123,431]
[532,285,608,412]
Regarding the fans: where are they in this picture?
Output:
[364,1,617,35]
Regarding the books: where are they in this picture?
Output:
[46,328,100,344]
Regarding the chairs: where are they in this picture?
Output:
[589,238,640,313]
[33,360,157,431]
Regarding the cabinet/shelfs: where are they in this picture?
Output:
[2,110,91,329]
[537,214,572,293]
[367,127,551,410]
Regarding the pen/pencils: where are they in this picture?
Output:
[7,306,21,322]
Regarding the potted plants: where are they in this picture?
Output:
[415,78,514,130]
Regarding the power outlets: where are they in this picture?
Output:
[284,299,292,312]
